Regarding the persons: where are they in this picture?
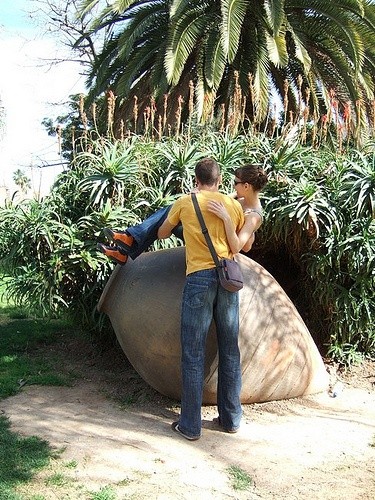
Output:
[97,164,267,266]
[157,158,255,441]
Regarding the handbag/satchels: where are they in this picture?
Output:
[217,258,244,293]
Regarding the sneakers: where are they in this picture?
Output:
[98,243,128,266]
[218,416,239,433]
[172,422,200,441]
[105,228,133,252]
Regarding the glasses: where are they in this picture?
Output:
[234,180,245,185]
